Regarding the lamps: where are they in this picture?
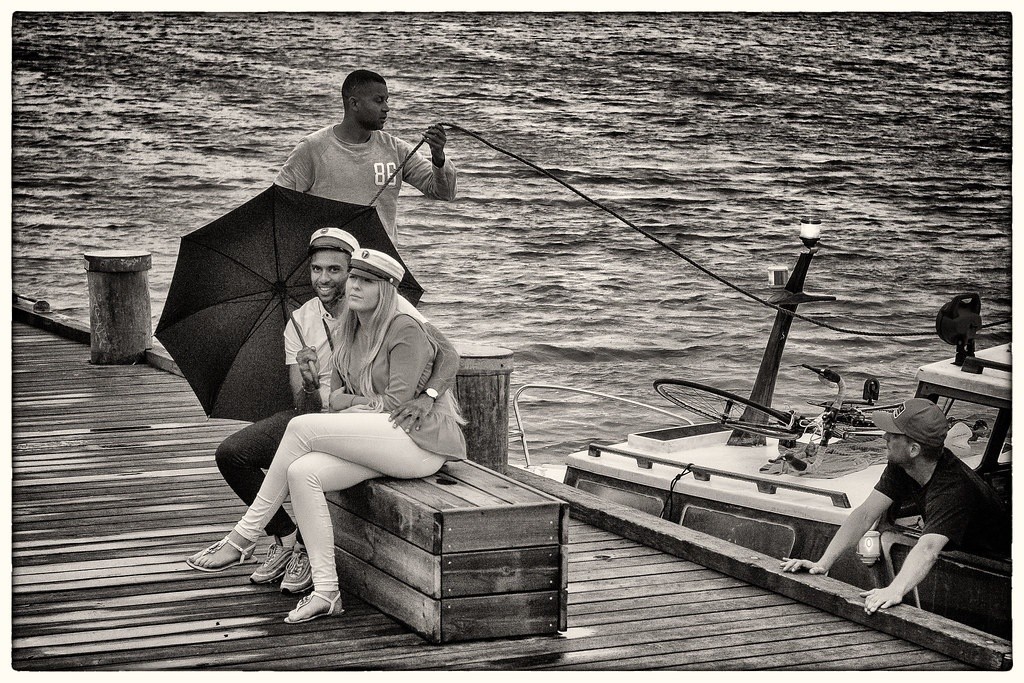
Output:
[935,294,983,366]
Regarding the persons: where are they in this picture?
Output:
[780,397,1011,615]
[216,227,459,595]
[275,69,457,246]
[186,248,466,623]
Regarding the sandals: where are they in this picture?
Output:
[185,535,259,572]
[282,591,345,623]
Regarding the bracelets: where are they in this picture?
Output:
[301,381,318,394]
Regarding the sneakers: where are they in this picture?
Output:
[250,542,295,584]
[279,544,314,593]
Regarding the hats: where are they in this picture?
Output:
[873,398,949,448]
[348,248,405,290]
[307,227,360,256]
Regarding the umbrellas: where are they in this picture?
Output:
[153,182,427,425]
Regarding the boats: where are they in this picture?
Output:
[512,216,1013,634]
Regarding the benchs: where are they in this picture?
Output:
[325,460,568,645]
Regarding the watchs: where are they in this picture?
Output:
[418,388,440,403]
